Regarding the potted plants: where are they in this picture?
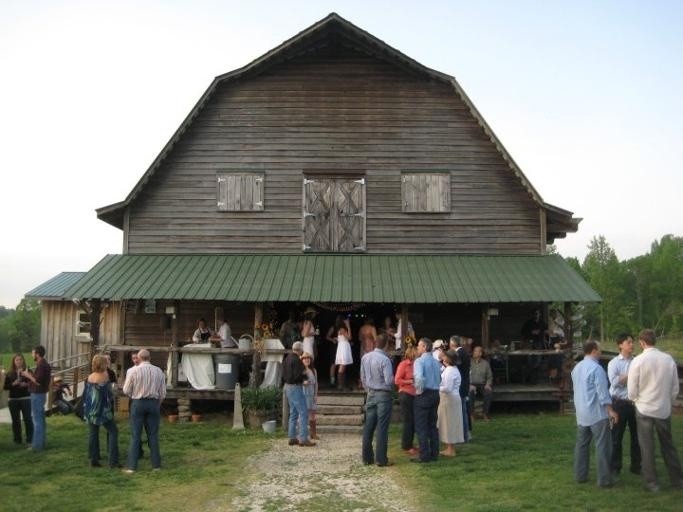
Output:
[258,384,280,433]
[240,386,282,430]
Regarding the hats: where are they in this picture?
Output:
[54,376,63,382]
[438,349,458,365]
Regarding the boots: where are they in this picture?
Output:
[337,371,352,391]
[309,419,320,440]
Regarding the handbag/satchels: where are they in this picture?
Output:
[75,400,82,417]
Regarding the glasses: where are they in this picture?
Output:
[304,307,317,314]
[300,352,314,361]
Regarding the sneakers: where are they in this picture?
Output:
[440,450,456,457]
[288,438,299,445]
[299,440,316,446]
[407,448,419,454]
[410,457,425,463]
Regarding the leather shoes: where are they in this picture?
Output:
[378,462,393,466]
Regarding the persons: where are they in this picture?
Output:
[191,317,213,344]
[86,350,119,461]
[626,328,683,492]
[608,332,642,477]
[569,339,620,488]
[211,310,237,349]
[81,354,125,470]
[280,306,494,468]
[122,347,167,475]
[124,349,152,459]
[51,375,75,415]
[549,308,565,338]
[3,352,34,448]
[19,344,51,452]
[519,308,544,386]
